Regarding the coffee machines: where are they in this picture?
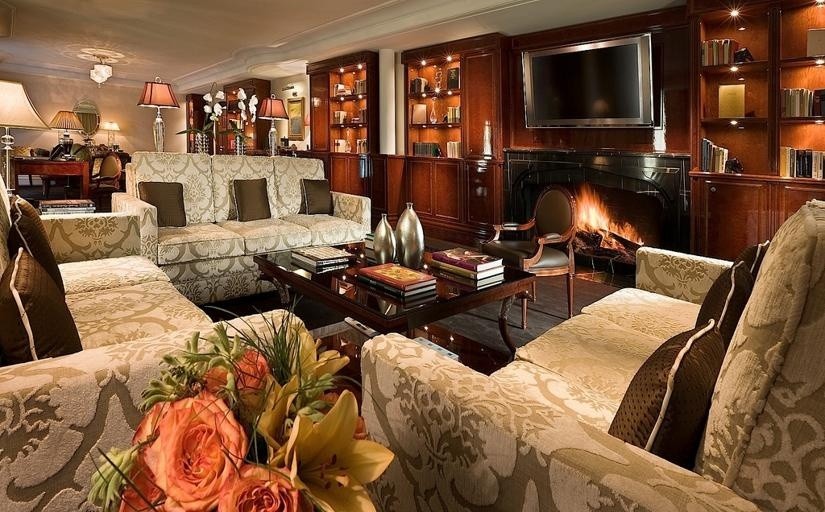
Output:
[280,138,288,149]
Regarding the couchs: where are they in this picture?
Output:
[108,149,373,306]
[358,196,825,512]
[0,176,314,512]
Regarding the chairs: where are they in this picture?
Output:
[479,183,578,332]
[0,146,126,200]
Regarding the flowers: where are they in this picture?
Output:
[217,87,259,140]
[175,81,225,141]
[82,290,399,511]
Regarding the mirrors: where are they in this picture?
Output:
[74,102,100,136]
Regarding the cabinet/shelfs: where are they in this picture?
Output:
[186,93,213,154]
[404,51,461,244]
[689,0,772,265]
[329,59,379,220]
[215,79,271,154]
[309,66,329,191]
[773,1,825,238]
[463,44,508,249]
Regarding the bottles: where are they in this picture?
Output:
[373,214,397,266]
[482,120,492,159]
[429,99,438,124]
[653,100,667,154]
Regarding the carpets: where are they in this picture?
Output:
[422,236,622,357]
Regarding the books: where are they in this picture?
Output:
[699,137,729,174]
[780,146,824,181]
[411,104,428,125]
[701,38,754,67]
[446,140,461,159]
[413,141,440,158]
[806,28,825,58]
[779,88,825,118]
[718,84,745,118]
[410,78,427,93]
[447,106,461,124]
[290,245,506,309]
[38,199,97,215]
[332,79,366,154]
[447,67,460,90]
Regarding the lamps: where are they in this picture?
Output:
[0,76,53,199]
[88,52,122,90]
[45,110,87,161]
[258,91,292,157]
[98,121,124,155]
[137,75,182,152]
[302,113,311,150]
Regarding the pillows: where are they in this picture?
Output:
[693,237,771,354]
[7,188,69,300]
[606,316,725,461]
[232,177,273,222]
[0,245,83,367]
[138,181,187,228]
[300,177,334,215]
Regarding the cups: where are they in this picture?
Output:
[111,144,119,152]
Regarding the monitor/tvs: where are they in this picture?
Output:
[522,32,655,131]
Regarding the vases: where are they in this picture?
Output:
[234,134,245,154]
[370,211,398,266]
[195,130,210,153]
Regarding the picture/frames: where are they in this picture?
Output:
[286,96,305,142]
